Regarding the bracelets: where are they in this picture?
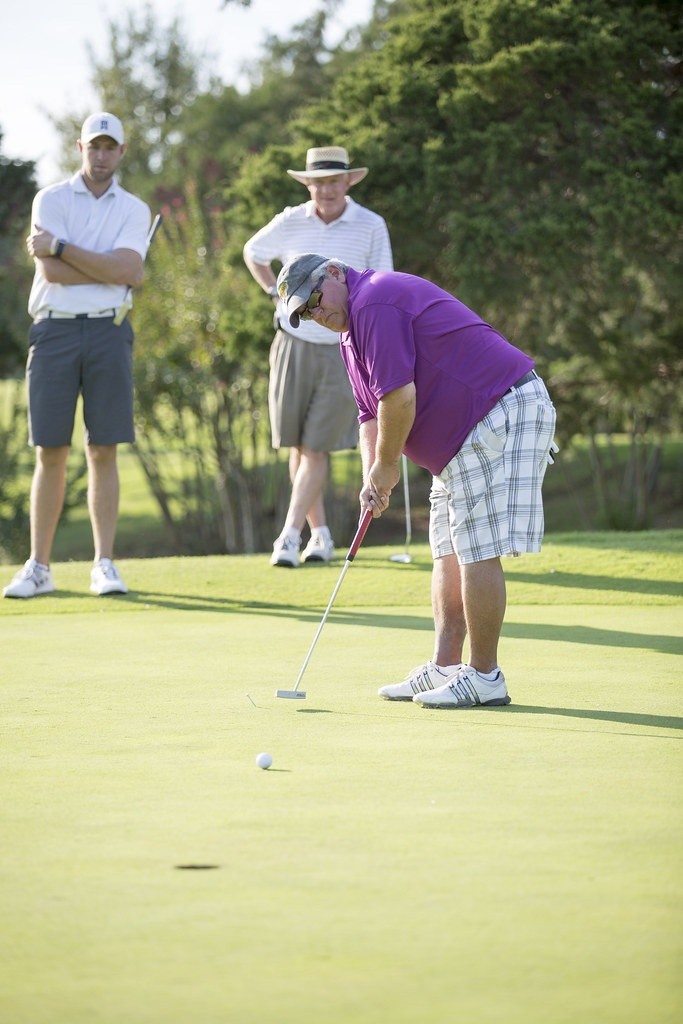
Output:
[51,237,58,255]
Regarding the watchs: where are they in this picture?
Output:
[269,287,277,299]
[55,239,66,257]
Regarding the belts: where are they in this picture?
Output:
[35,308,123,320]
[502,369,540,392]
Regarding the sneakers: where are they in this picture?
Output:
[90,560,126,596]
[411,667,511,709]
[301,533,338,564]
[268,534,301,568]
[2,561,57,598]
[376,661,465,702]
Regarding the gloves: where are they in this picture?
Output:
[547,442,560,467]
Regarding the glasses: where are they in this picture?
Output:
[300,274,324,324]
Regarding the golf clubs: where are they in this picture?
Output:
[113,213,163,326]
[388,453,413,563]
[275,495,374,699]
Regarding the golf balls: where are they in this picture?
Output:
[256,752,272,769]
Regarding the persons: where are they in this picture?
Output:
[276,253,555,708]
[243,146,394,567]
[4,111,149,600]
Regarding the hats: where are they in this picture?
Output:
[81,112,127,147]
[275,253,330,329]
[286,146,369,188]
